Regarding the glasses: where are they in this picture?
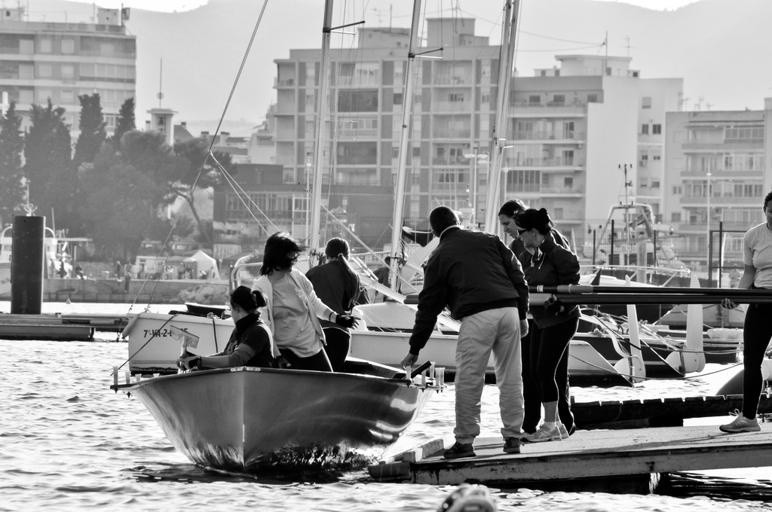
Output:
[517,229,527,235]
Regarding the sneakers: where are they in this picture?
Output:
[521,423,570,442]
[444,442,476,458]
[503,437,520,452]
[719,413,762,432]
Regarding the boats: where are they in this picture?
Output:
[2,224,230,306]
[111,358,448,470]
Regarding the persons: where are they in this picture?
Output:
[513,208,582,443]
[719,191,772,435]
[399,204,530,460]
[367,255,390,303]
[305,237,361,370]
[497,197,577,436]
[250,229,361,373]
[173,284,274,367]
[48,256,235,295]
